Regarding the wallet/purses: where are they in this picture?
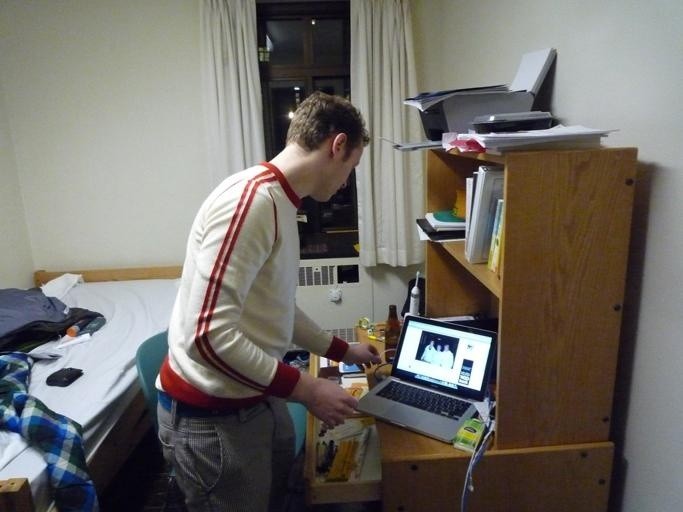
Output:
[45,366,84,388]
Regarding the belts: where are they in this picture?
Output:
[156,389,257,420]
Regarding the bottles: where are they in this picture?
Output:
[384,305,402,363]
[66,315,105,337]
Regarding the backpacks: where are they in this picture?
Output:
[0,286,93,352]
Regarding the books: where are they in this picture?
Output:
[402,84,509,113]
[415,166,505,281]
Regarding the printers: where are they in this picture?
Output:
[419,92,535,141]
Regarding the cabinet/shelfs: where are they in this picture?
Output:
[418,139,639,452]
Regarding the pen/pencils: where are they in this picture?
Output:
[316,440,338,472]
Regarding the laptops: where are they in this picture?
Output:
[354,313,497,444]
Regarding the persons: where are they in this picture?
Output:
[154,91,382,512]
[420,339,454,370]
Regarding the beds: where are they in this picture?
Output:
[1,261,181,511]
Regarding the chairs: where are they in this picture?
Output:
[133,327,308,510]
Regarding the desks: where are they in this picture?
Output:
[300,319,621,512]
